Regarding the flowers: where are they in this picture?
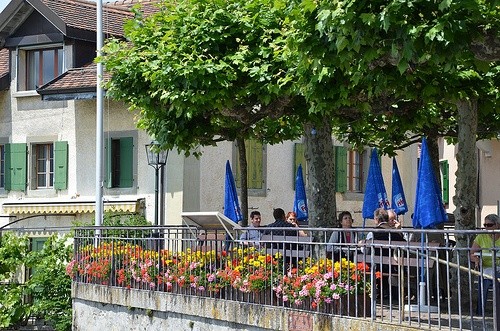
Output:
[64,240,383,308]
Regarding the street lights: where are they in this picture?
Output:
[144,140,169,225]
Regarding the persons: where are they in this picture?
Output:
[263,209,303,265]
[239,212,264,249]
[383,209,403,235]
[469,215,500,317]
[286,212,308,237]
[357,208,408,300]
[327,212,362,262]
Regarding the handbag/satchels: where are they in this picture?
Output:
[327,228,345,263]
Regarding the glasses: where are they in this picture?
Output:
[483,223,495,228]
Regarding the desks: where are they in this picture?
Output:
[472,251,500,260]
[334,246,362,263]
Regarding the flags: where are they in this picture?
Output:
[412,136,449,231]
[362,147,391,220]
[391,157,408,216]
[224,160,244,256]
[293,164,308,222]
